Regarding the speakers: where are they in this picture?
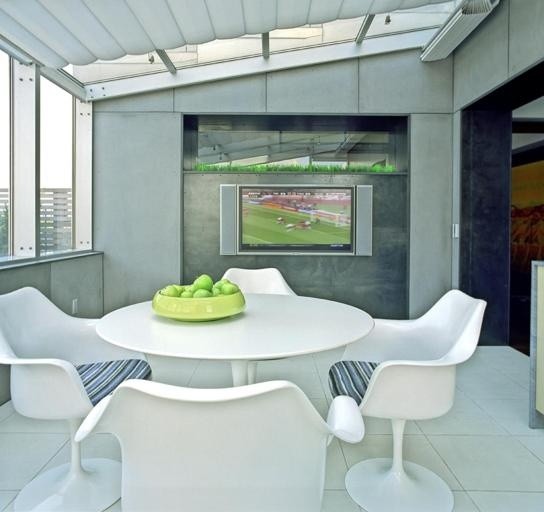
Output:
[219,183,236,255]
[356,184,373,257]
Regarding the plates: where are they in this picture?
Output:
[153,287,246,322]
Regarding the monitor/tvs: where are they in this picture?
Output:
[236,184,356,256]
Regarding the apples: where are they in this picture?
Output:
[214,278,228,290]
[161,285,177,297]
[193,289,212,297]
[181,285,193,297]
[193,274,213,291]
[211,287,223,295]
[222,284,238,294]
[173,284,185,297]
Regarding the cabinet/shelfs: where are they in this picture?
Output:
[529,261,543,428]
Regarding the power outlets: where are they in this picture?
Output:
[72,298,78,314]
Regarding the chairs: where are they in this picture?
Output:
[0,286,151,512]
[328,290,488,512]
[73,379,365,512]
[221,268,298,295]
